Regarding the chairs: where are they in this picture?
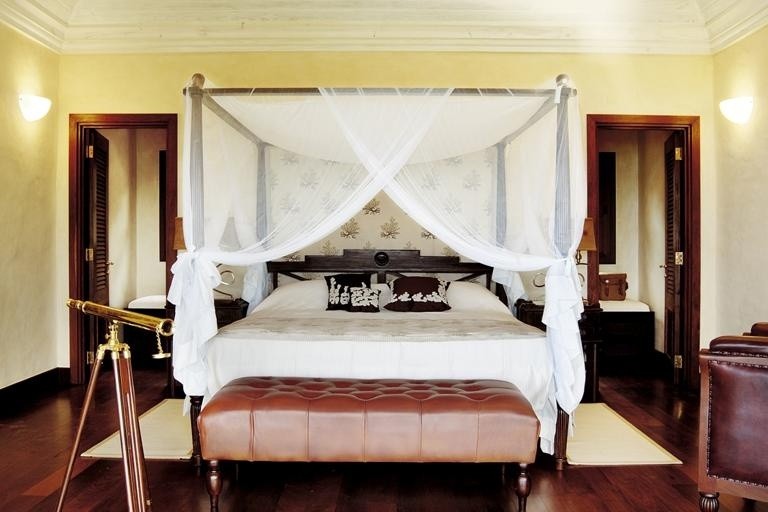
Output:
[698,321,768,512]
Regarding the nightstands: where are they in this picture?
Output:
[513,297,606,402]
[173,296,247,395]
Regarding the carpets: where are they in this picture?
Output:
[80,398,193,460]
[565,402,685,466]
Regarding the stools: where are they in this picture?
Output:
[122,295,169,372]
[599,298,656,380]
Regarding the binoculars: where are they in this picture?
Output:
[66,298,173,337]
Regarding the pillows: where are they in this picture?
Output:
[348,285,381,312]
[322,273,372,312]
[250,279,510,314]
[383,277,452,312]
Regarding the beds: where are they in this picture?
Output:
[198,249,569,477]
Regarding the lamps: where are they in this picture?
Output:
[575,218,597,267]
[173,216,186,253]
[219,217,242,249]
[19,93,51,122]
[720,95,751,124]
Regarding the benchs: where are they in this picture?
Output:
[194,377,541,512]
